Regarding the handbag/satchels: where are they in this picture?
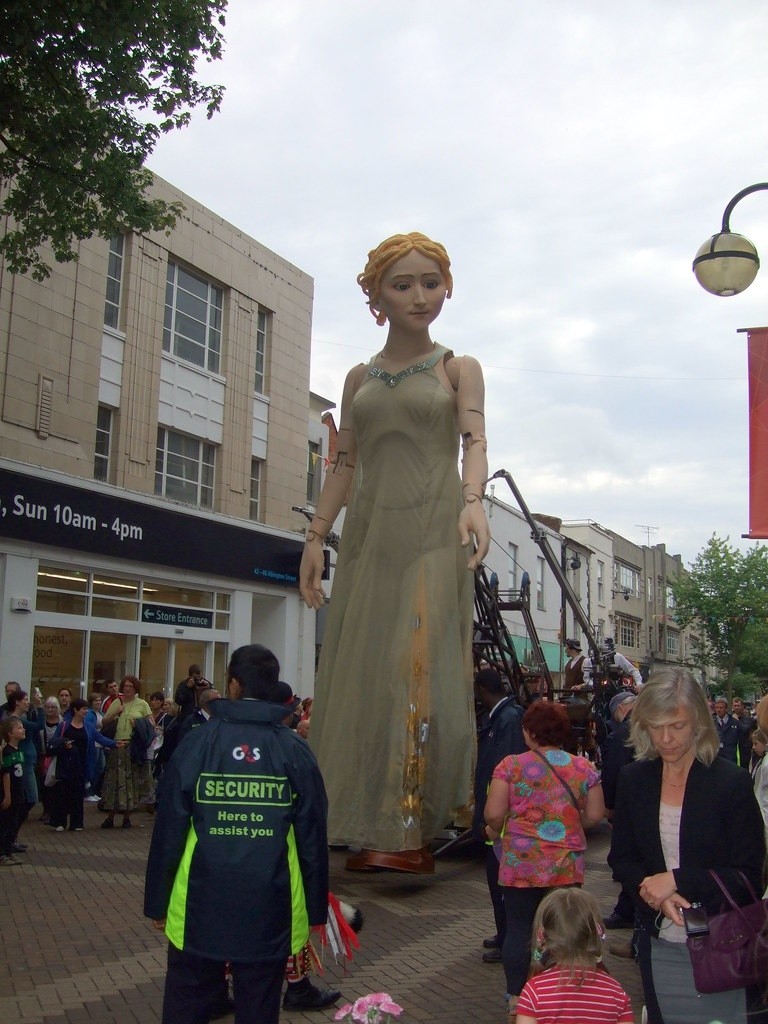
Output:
[144,725,164,761]
[37,754,61,786]
[685,869,768,993]
[100,698,123,740]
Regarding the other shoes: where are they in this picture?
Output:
[12,844,28,852]
[609,942,632,958]
[39,812,51,824]
[101,818,114,829]
[483,936,502,947]
[122,819,131,829]
[83,795,102,801]
[75,828,83,831]
[482,950,504,962]
[508,995,519,1018]
[56,826,64,832]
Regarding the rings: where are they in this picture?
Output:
[647,900,654,904]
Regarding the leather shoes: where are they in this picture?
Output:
[282,974,342,1011]
[209,1000,235,1019]
[602,913,636,930]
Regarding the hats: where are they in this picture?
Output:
[271,682,302,707]
[568,639,582,650]
[474,667,502,691]
[609,692,635,721]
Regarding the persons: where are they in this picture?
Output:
[607,667,768,1024]
[219,680,342,1017]
[299,233,488,875]
[517,888,633,1024]
[484,702,605,1017]
[472,638,768,963]
[143,645,328,1024]
[0,663,313,866]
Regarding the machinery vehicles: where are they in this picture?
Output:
[292,470,632,856]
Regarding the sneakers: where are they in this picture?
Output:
[9,854,24,864]
[0,855,15,866]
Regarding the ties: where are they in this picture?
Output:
[721,719,724,727]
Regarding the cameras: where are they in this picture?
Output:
[193,677,203,687]
[681,902,710,938]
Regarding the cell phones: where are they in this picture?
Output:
[34,687,43,698]
